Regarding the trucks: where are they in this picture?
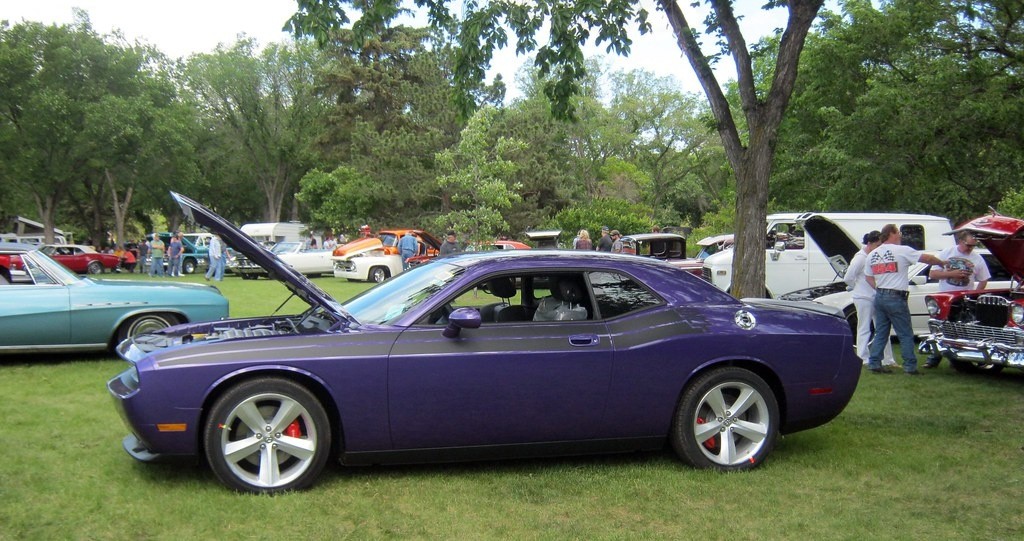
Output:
[231,222,322,276]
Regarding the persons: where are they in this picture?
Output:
[922,229,991,368]
[103,230,232,283]
[323,233,338,251]
[843,231,901,369]
[573,225,660,256]
[397,231,418,273]
[439,230,462,258]
[864,224,950,376]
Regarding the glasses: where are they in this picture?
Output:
[961,240,975,247]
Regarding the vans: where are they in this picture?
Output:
[701,213,957,300]
[0,233,68,253]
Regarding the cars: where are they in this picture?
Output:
[238,239,344,279]
[917,204,1024,370]
[135,232,237,274]
[182,234,214,247]
[9,243,123,275]
[619,232,703,276]
[776,212,1024,349]
[104,190,864,496]
[665,234,734,277]
[332,230,531,282]
[0,243,230,359]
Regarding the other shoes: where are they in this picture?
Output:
[904,371,919,375]
[921,363,933,368]
[872,368,892,374]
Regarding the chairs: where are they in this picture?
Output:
[487,277,527,322]
[533,277,587,321]
